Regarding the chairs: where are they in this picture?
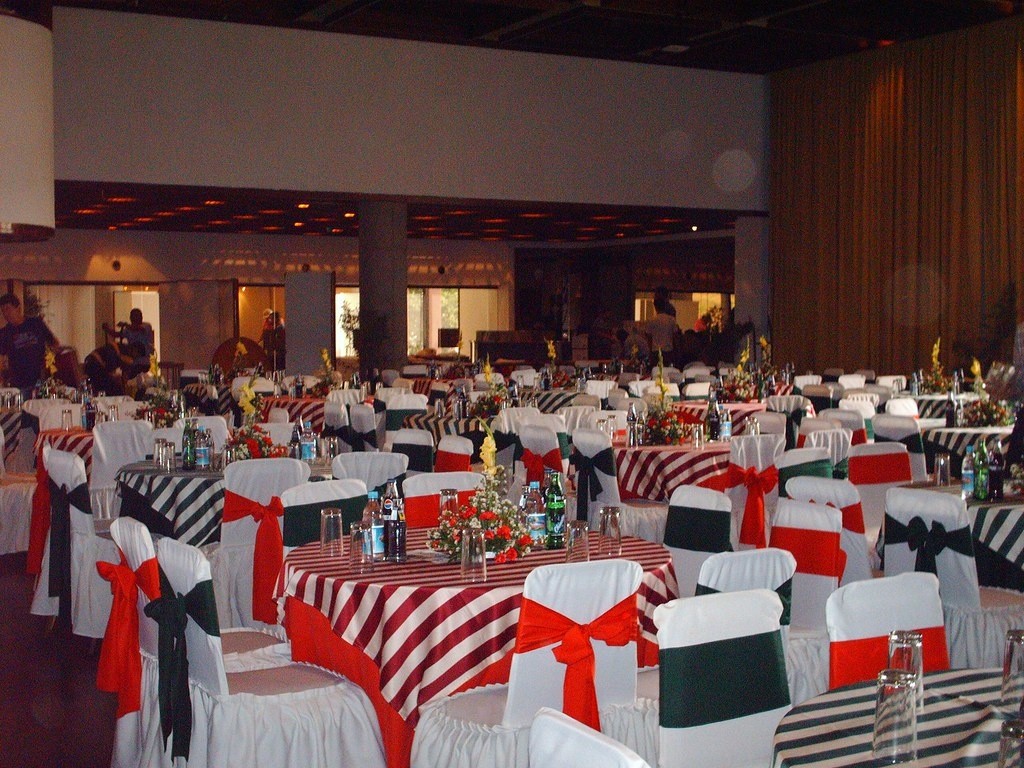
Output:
[0,358,1024,768]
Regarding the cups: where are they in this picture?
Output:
[451,398,459,418]
[745,416,758,436]
[529,396,538,408]
[517,375,524,389]
[465,401,471,417]
[690,422,704,450]
[534,377,540,391]
[3,391,179,472]
[933,451,950,487]
[198,371,206,384]
[434,398,444,415]
[318,484,1024,768]
[747,422,760,436]
[323,436,338,467]
[607,415,618,440]
[499,401,507,411]
[595,419,610,436]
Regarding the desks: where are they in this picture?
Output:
[894,392,990,429]
[400,413,493,457]
[272,516,681,736]
[115,448,335,549]
[185,381,241,424]
[28,426,97,576]
[925,487,1023,574]
[515,388,580,414]
[255,393,326,435]
[672,400,763,439]
[928,427,1012,478]
[774,660,1024,766]
[616,443,732,502]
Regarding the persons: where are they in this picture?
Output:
[257,312,286,370]
[615,295,712,368]
[103,310,153,381]
[0,295,60,400]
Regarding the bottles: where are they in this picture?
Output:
[546,471,565,549]
[523,480,546,551]
[387,497,407,562]
[540,468,552,508]
[423,359,791,460]
[379,474,400,561]
[361,491,385,562]
[182,363,384,472]
[908,367,1005,502]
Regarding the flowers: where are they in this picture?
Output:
[641,347,693,445]
[475,350,512,417]
[538,340,570,389]
[314,346,341,397]
[921,336,959,394]
[426,412,535,565]
[135,350,185,432]
[39,347,72,403]
[215,338,286,463]
[966,357,1013,428]
[721,338,774,402]
